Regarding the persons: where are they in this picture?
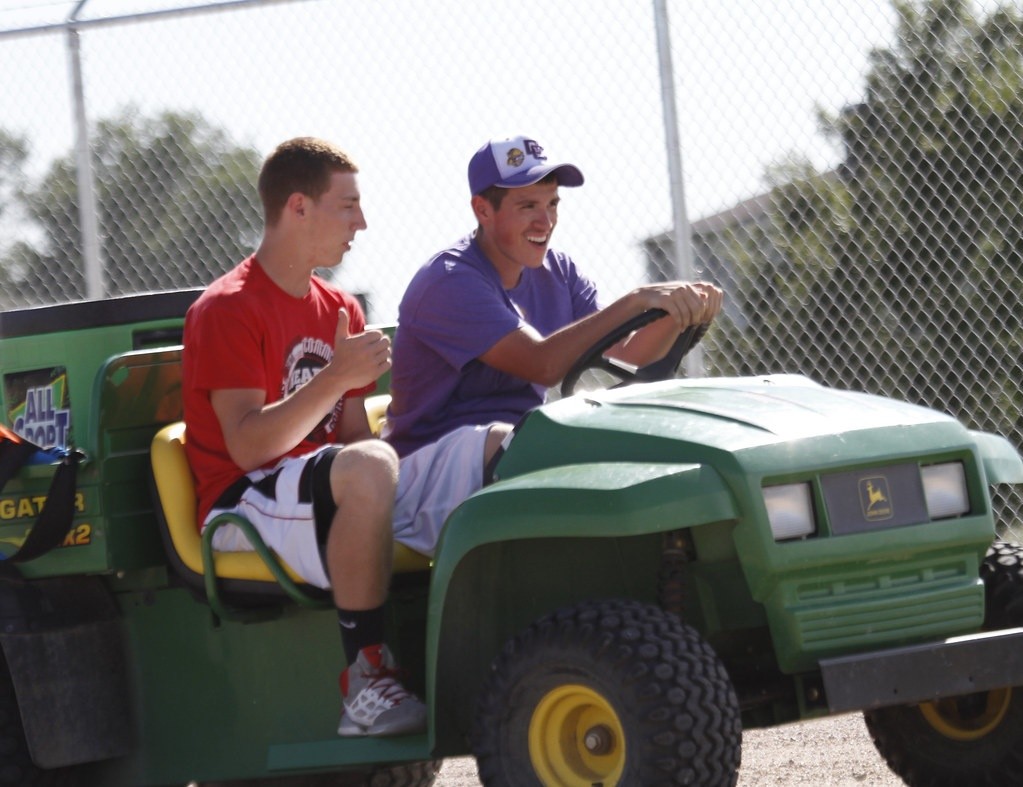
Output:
[379,134,723,559]
[180,137,428,738]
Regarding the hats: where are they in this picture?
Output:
[469,136,585,196]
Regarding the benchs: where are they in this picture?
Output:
[150,391,434,596]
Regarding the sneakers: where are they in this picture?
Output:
[338,644,426,737]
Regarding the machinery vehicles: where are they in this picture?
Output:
[0,284,1022,787]
